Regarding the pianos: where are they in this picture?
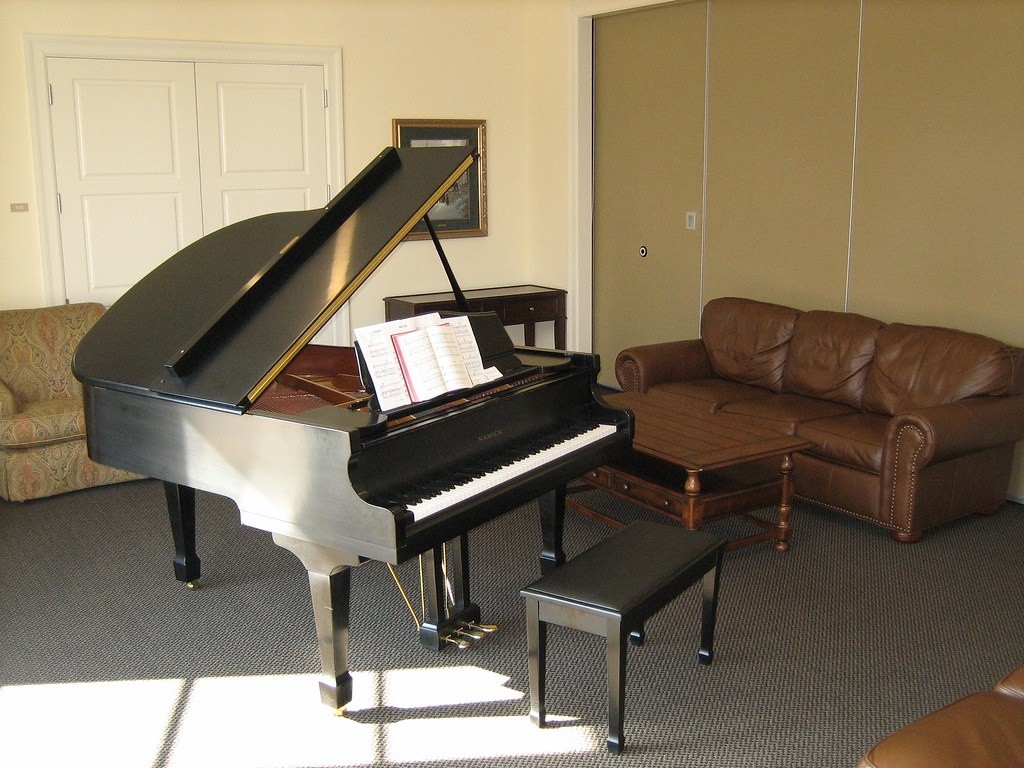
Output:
[70,145,639,721]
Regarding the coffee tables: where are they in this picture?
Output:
[563,390,816,552]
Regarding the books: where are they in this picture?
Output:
[391,322,474,404]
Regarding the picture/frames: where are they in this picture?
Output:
[391,118,488,241]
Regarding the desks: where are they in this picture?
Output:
[383,285,568,350]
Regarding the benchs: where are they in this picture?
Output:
[519,519,730,755]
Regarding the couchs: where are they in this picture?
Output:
[858,665,1024,768]
[615,297,1024,543]
[0,302,152,502]
